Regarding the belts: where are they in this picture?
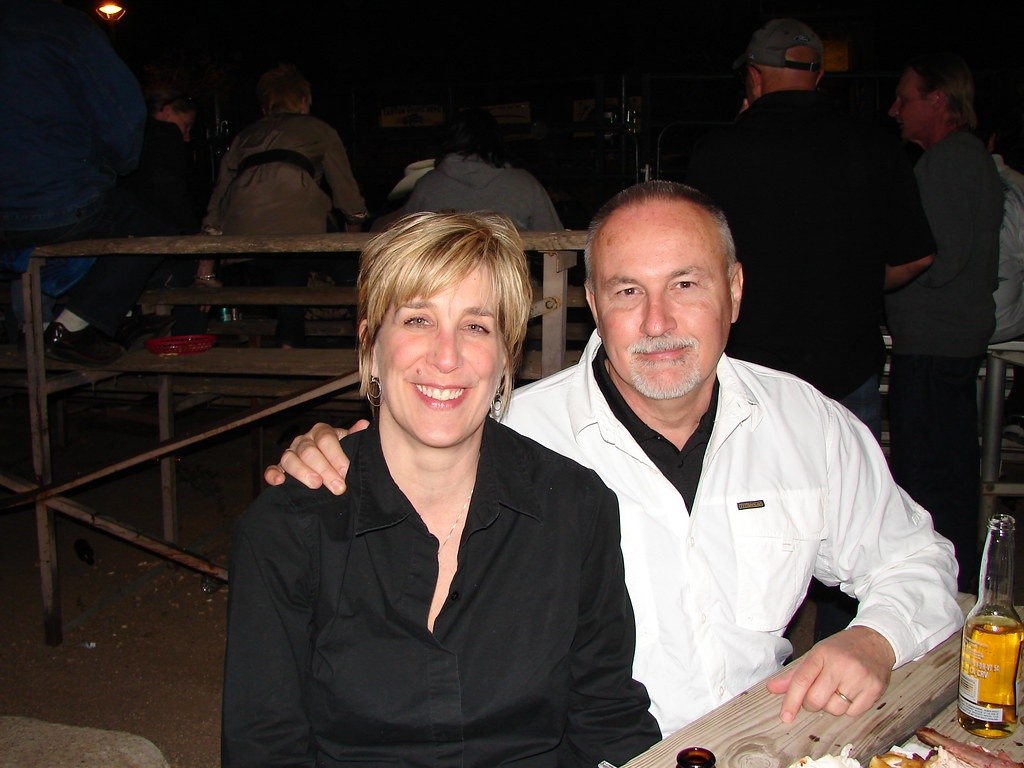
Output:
[238,149,316,179]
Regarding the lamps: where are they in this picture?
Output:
[96,1,126,26]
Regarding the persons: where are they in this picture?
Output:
[265,180,965,740]
[882,54,1004,593]
[0,0,167,365]
[114,93,228,336]
[404,108,564,231]
[683,19,938,439]
[191,63,369,350]
[220,210,663,768]
[966,112,1024,444]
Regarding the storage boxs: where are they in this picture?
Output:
[378,103,443,129]
[487,101,532,145]
[575,96,618,138]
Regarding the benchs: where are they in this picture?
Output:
[0,230,1024,653]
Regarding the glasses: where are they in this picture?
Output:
[734,63,761,83]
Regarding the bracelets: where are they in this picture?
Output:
[193,274,216,280]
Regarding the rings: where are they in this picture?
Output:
[835,690,853,705]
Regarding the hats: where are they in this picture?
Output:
[734,18,823,72]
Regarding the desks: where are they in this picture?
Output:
[619,591,1024,768]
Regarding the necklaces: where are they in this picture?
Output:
[437,488,473,554]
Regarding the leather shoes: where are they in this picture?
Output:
[17,321,122,362]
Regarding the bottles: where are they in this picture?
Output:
[675,746,717,768]
[626,98,643,135]
[955,515,1024,738]
